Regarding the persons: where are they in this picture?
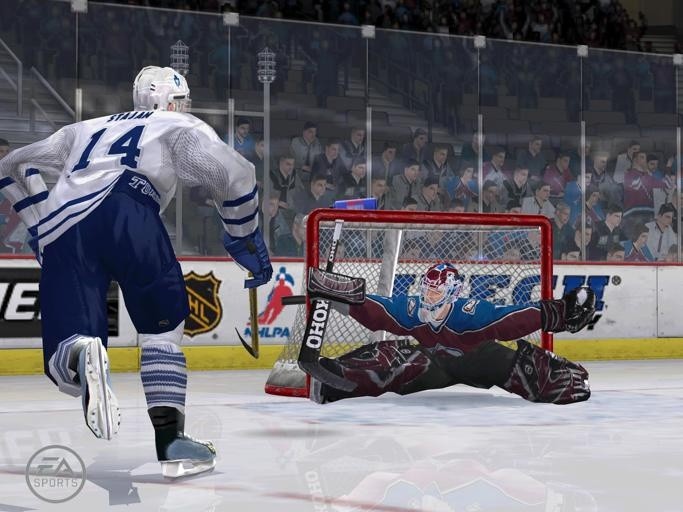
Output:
[307,263,596,406]
[1,64,274,480]
[0,0,679,265]
